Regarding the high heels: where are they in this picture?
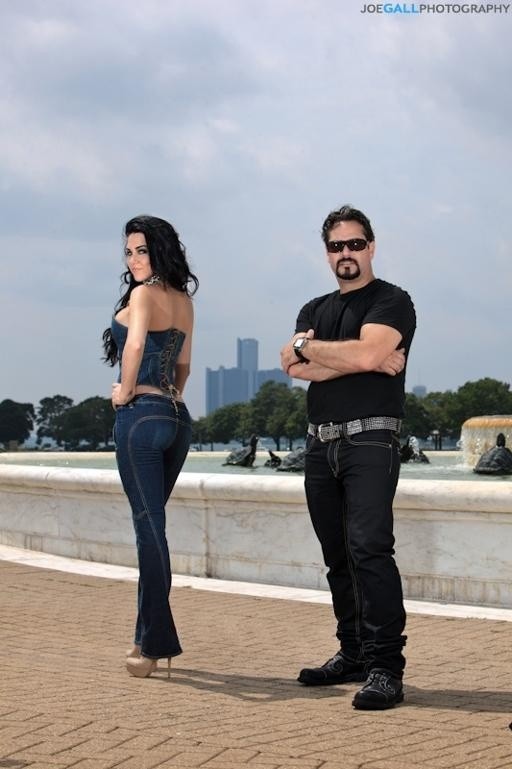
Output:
[125,645,172,677]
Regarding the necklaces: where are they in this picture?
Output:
[144,276,161,285]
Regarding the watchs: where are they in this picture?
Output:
[294,336,310,364]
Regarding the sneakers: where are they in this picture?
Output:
[297,653,373,687]
[349,668,404,709]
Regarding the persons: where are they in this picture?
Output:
[101,212,194,682]
[278,204,417,710]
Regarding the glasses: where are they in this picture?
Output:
[328,238,366,252]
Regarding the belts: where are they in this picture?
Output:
[307,416,402,443]
